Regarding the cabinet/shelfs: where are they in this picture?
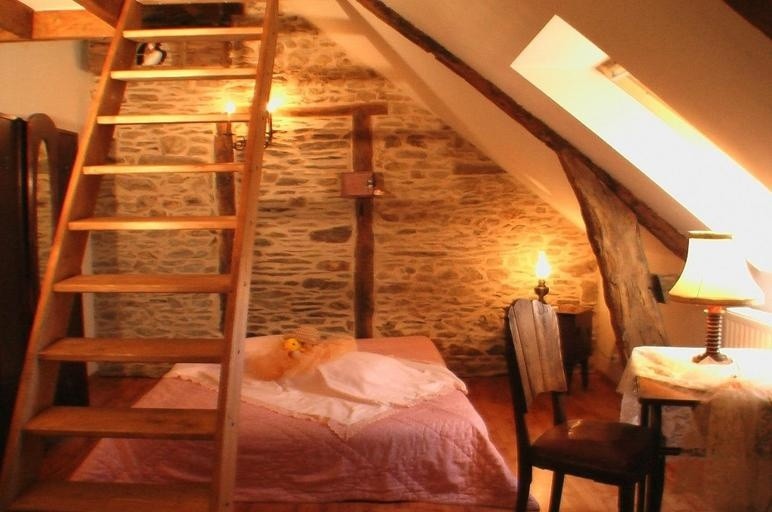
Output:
[551,304,593,395]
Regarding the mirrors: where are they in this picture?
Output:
[24,114,63,303]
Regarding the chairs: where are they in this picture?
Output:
[500,297,664,512]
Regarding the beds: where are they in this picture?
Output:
[73,333,507,512]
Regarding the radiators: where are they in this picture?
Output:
[721,305,771,352]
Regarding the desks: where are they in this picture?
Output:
[626,347,772,511]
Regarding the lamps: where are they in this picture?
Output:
[660,230,767,368]
